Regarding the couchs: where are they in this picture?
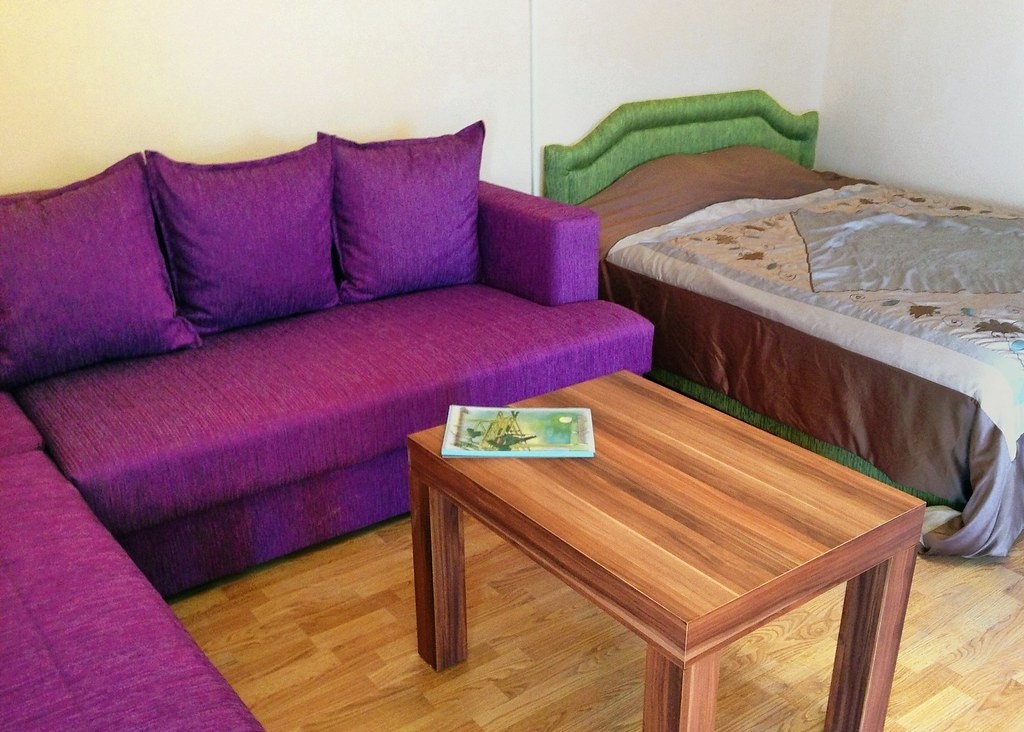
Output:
[0,392,268,732]
[0,172,660,604]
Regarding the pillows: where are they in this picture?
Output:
[0,152,202,391]
[144,134,344,333]
[316,119,486,301]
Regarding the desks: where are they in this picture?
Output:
[410,368,925,732]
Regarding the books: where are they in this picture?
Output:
[441,404,596,458]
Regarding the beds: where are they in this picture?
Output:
[540,89,1024,561]
